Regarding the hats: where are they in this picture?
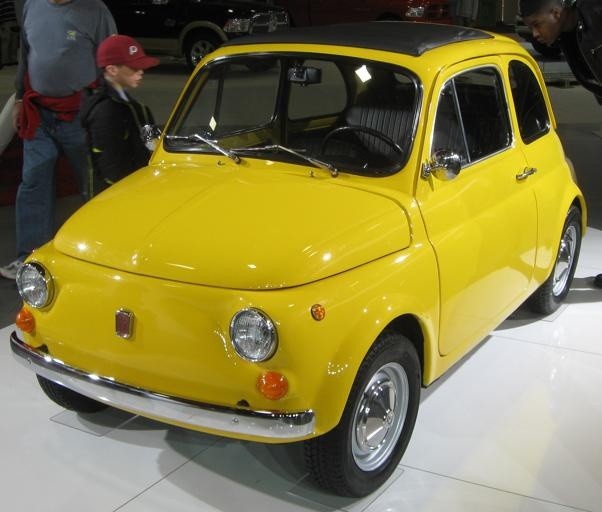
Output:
[94,33,161,70]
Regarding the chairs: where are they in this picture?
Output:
[339,82,507,170]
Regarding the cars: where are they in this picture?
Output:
[106,0,291,75]
[10,17,589,499]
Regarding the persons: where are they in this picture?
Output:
[520,0,602,289]
[77,34,158,201]
[0,2,121,280]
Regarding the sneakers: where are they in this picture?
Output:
[0,259,25,281]
